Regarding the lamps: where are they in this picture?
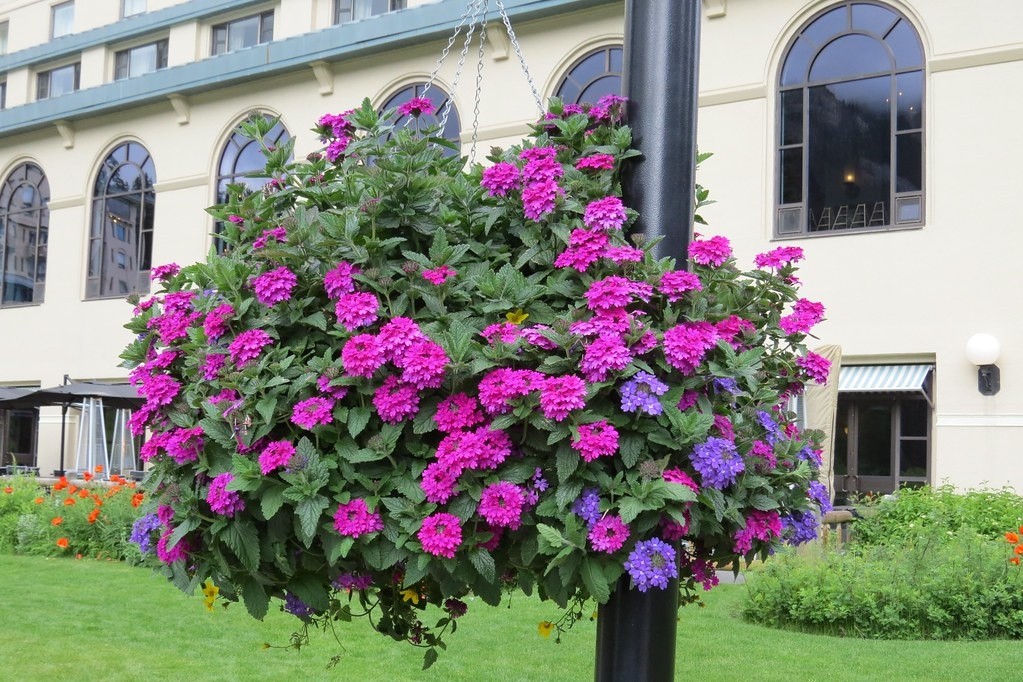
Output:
[964,332,1003,398]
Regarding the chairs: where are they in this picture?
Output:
[0,463,154,490]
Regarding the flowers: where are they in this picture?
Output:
[121,86,828,666]
